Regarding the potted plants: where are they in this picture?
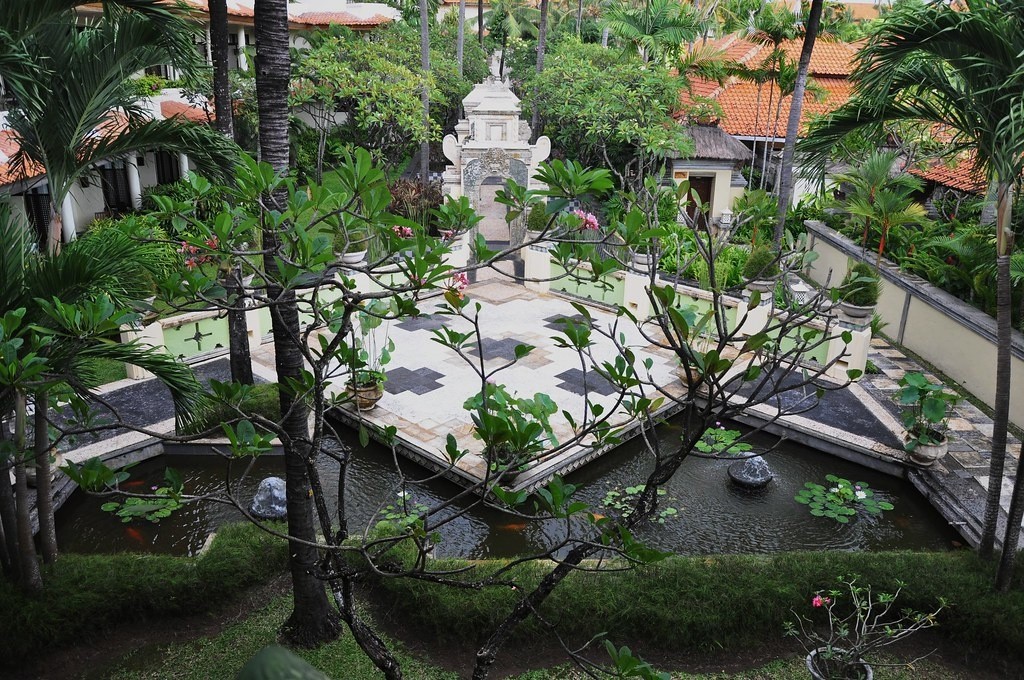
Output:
[11,417,62,490]
[345,315,388,411]
[333,227,368,264]
[478,390,535,483]
[116,262,157,319]
[525,201,560,239]
[838,261,880,316]
[893,371,959,467]
[742,243,781,292]
[436,198,469,241]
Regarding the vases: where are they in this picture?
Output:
[806,646,875,680]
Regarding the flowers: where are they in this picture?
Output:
[812,596,831,608]
